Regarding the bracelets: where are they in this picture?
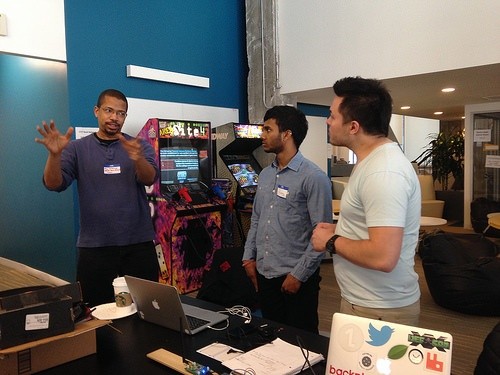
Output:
[242,261,251,266]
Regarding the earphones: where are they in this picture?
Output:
[227,306,252,323]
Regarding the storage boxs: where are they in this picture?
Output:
[0,282,110,375]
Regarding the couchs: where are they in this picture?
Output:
[421,232,500,317]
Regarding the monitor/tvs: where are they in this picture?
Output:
[159,147,199,184]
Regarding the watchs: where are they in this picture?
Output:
[326,234,341,255]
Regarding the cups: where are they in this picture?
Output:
[112,277,132,308]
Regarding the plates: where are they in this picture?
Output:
[90,303,138,320]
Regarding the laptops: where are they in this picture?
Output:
[125,275,229,334]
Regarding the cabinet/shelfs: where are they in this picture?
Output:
[462,102,500,230]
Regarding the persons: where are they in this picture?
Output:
[32,88,159,305]
[311,76,422,328]
[241,106,333,336]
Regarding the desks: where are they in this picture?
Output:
[35,295,330,375]
